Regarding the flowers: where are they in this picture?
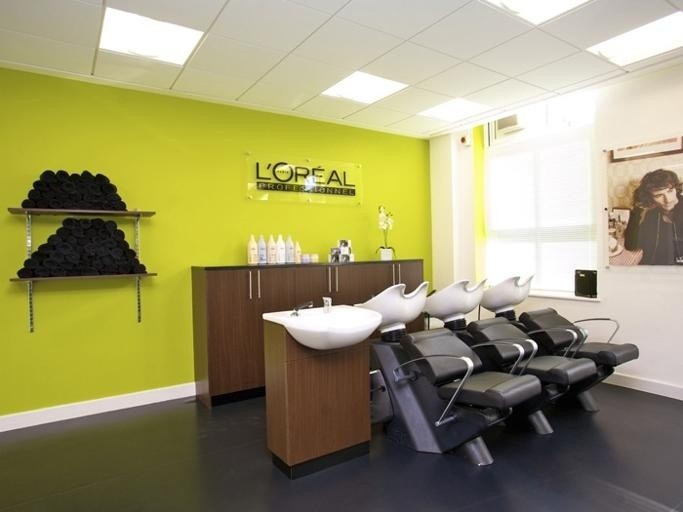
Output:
[373,204,399,257]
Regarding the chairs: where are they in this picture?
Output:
[393,307,640,426]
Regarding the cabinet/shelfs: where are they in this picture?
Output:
[5,205,157,280]
[190,261,424,409]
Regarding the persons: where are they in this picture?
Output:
[623,167,682,265]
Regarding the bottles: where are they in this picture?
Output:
[248,233,302,265]
[608,212,616,229]
[615,214,623,237]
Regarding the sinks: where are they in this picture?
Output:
[480,274,535,314]
[261,302,384,350]
[425,277,487,321]
[352,281,430,335]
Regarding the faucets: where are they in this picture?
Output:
[292,300,314,311]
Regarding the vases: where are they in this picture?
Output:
[378,248,393,263]
